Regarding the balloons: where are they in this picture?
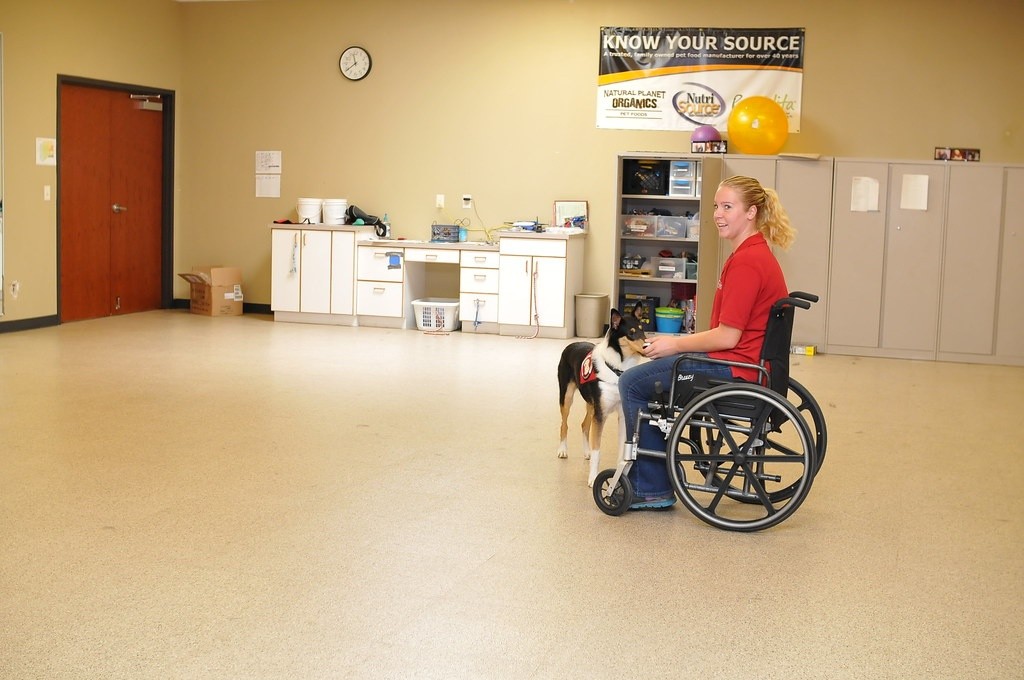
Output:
[691,127,721,141]
[728,96,789,154]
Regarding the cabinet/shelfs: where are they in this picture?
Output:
[499,227,587,338]
[611,151,1024,369]
[270,222,384,324]
[356,239,500,333]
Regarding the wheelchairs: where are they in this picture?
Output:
[591,290,827,534]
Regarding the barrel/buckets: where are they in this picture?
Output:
[295,198,323,224]
[323,199,349,224]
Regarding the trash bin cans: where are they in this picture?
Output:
[575,293,609,338]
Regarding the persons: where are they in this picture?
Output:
[619,175,795,508]
[696,142,725,152]
[938,150,979,160]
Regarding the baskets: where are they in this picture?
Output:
[412,296,462,332]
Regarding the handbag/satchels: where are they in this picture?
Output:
[346,204,380,225]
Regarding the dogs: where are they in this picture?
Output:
[557,300,649,490]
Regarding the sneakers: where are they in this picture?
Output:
[607,489,677,510]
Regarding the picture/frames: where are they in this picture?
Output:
[553,199,589,227]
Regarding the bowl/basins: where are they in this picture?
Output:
[655,307,684,332]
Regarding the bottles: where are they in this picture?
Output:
[383,213,391,238]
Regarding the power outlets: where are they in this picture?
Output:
[463,195,471,209]
[436,195,445,208]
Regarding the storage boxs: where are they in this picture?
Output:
[686,220,699,239]
[669,161,697,181]
[619,297,660,332]
[696,179,702,198]
[621,214,657,238]
[177,265,244,317]
[410,297,460,332]
[686,263,697,279]
[650,256,686,280]
[620,255,646,269]
[669,283,696,300]
[791,344,817,356]
[624,162,666,194]
[657,216,688,238]
[668,180,696,197]
[696,162,702,179]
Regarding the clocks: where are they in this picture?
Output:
[339,46,372,81]
